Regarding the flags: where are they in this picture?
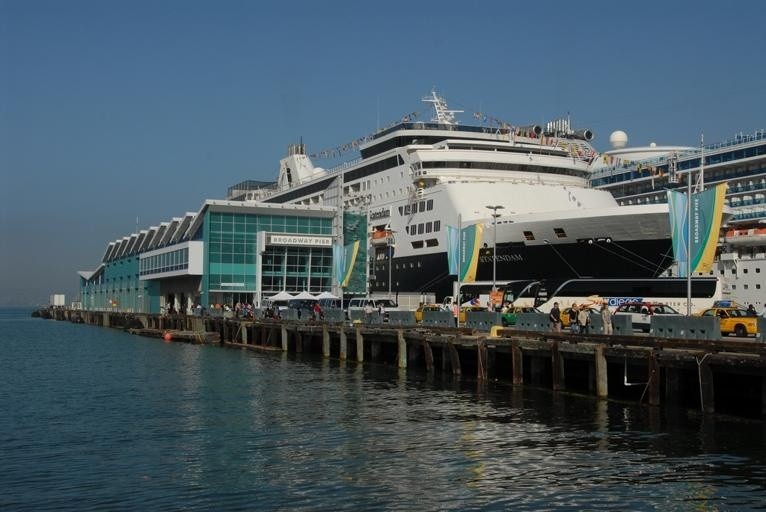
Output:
[473,110,682,189]
[302,110,418,161]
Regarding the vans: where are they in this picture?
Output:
[348,297,404,321]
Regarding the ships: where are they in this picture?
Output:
[226,85,733,309]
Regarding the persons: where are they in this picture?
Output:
[747,303,766,319]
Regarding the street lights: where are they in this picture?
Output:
[382,230,397,298]
[485,204,505,313]
